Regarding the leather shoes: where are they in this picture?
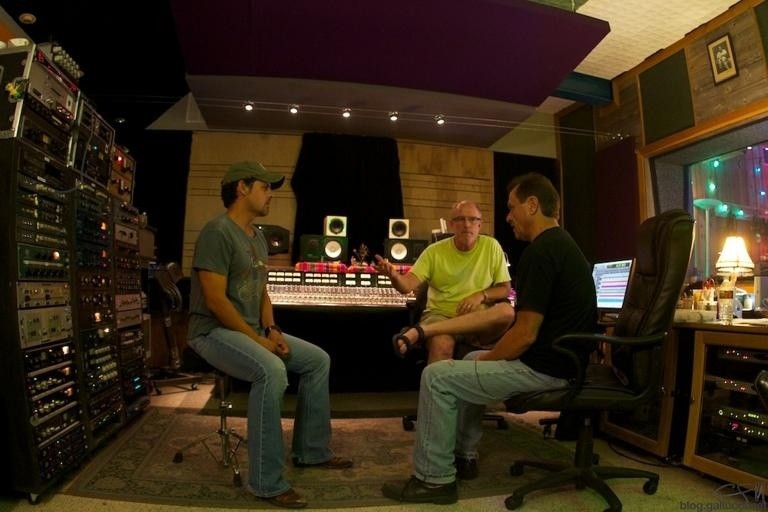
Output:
[267,489,308,508]
[457,458,478,478]
[382,476,457,504]
[299,457,353,469]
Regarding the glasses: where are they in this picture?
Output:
[452,215,481,223]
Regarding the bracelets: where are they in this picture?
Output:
[483,288,488,304]
[264,325,282,337]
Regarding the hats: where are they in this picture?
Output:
[221,161,285,189]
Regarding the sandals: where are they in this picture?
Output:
[392,325,424,353]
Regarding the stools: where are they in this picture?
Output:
[172,348,256,487]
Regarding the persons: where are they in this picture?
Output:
[381,173,597,505]
[186,161,354,508]
[371,198,515,361]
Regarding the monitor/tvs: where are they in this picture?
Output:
[590,259,636,311]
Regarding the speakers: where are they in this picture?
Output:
[389,219,410,239]
[249,222,291,256]
[385,238,428,266]
[299,235,348,264]
[323,215,347,238]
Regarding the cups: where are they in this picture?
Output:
[682,289,717,310]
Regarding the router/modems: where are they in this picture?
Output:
[2,39,153,503]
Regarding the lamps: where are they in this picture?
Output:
[715,235,755,297]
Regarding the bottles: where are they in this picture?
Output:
[743,293,753,310]
[718,276,733,325]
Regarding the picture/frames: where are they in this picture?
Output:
[706,34,739,86]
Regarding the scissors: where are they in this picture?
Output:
[704,279,714,289]
